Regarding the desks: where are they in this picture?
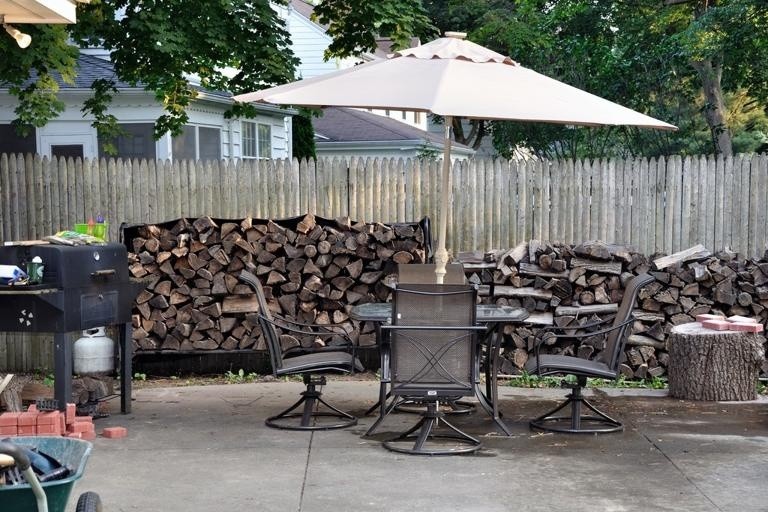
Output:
[348,303,530,436]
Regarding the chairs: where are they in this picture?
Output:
[523,271,656,434]
[379,282,488,455]
[393,263,478,417]
[238,268,366,433]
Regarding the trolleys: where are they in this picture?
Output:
[0,436,102,512]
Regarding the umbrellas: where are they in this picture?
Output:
[228,30,679,315]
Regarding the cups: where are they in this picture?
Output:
[27,262,45,284]
[75,223,88,234]
[94,223,105,238]
